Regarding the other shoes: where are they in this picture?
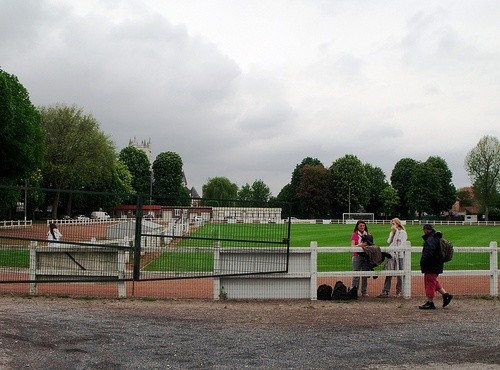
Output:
[442,292,453,307]
[396,294,400,298]
[418,301,435,309]
[362,293,370,297]
[378,293,390,298]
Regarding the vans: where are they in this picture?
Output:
[91,211,111,221]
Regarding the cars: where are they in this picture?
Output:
[74,214,90,222]
[62,215,72,223]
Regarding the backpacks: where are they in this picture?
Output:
[433,236,455,262]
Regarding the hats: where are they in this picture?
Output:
[423,224,435,230]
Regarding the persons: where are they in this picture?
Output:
[375,218,407,298]
[47,224,62,247]
[352,221,369,297]
[418,224,452,310]
